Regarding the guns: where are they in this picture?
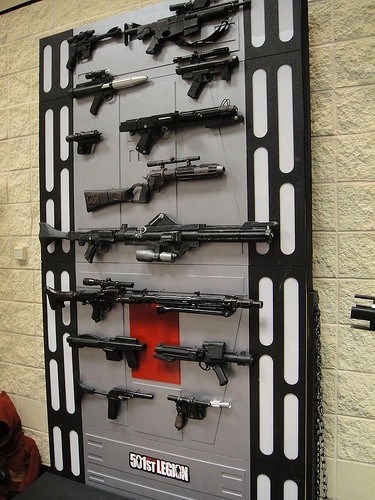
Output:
[38,212,280,265]
[66,26,121,70]
[65,129,103,155]
[173,46,240,99]
[119,99,246,155]
[68,67,150,114]
[167,394,232,433]
[152,340,253,386]
[122,0,250,54]
[72,375,154,421]
[83,155,227,213]
[44,277,264,326]
[66,333,147,369]
